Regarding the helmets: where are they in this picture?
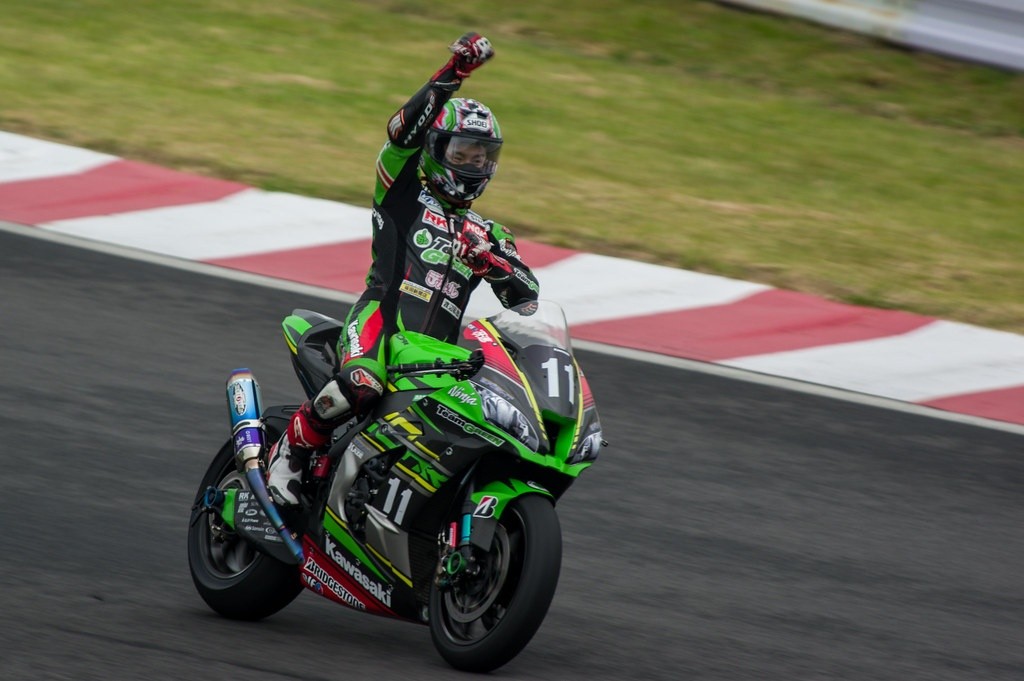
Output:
[419,98,504,202]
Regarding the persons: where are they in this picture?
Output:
[268,32,539,513]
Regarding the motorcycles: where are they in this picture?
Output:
[187,295,607,672]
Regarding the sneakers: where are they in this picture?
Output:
[267,399,333,508]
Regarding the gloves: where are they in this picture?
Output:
[453,230,491,265]
[447,33,494,78]
